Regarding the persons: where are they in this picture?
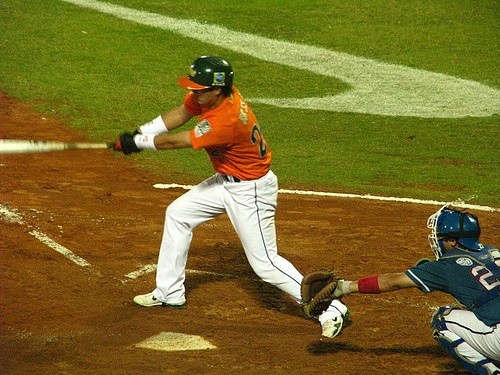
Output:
[301,207,500,375]
[117,55,351,336]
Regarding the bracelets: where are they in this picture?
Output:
[140,113,167,136]
[358,274,381,294]
[135,133,160,152]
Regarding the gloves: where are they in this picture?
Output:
[113,130,143,155]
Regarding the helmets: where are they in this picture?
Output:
[437,212,485,251]
[178,56,233,89]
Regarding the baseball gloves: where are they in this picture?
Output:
[301,271,343,317]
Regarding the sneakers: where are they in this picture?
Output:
[321,307,351,339]
[133,293,186,307]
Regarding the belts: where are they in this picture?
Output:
[221,174,241,183]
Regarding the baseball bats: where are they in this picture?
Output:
[0,138,116,155]
